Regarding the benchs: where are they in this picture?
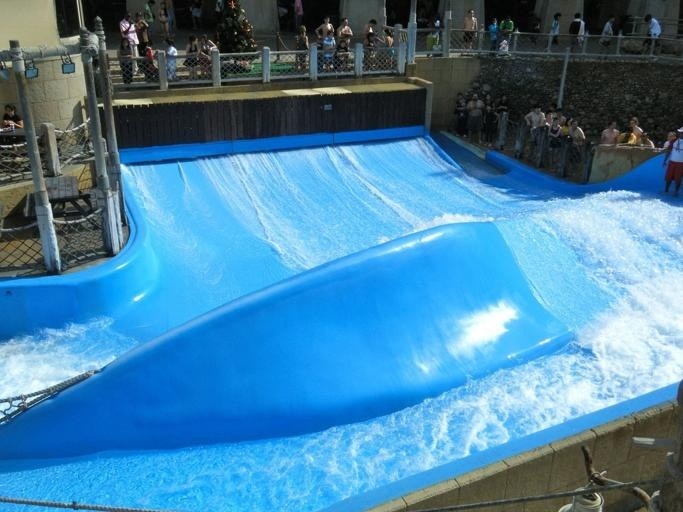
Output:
[25,192,37,220]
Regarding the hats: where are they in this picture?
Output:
[677,127,683,132]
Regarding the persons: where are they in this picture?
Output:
[463,9,479,51]
[433,16,441,45]
[487,18,499,51]
[452,91,655,174]
[661,127,683,195]
[664,131,677,150]
[363,18,376,40]
[598,14,615,55]
[362,32,376,58]
[643,14,661,53]
[546,12,561,54]
[116,1,355,83]
[500,17,513,47]
[569,12,586,54]
[1,103,26,131]
[383,28,394,67]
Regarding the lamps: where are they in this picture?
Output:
[0,53,75,81]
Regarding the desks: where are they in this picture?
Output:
[0,128,40,173]
[45,176,92,218]
